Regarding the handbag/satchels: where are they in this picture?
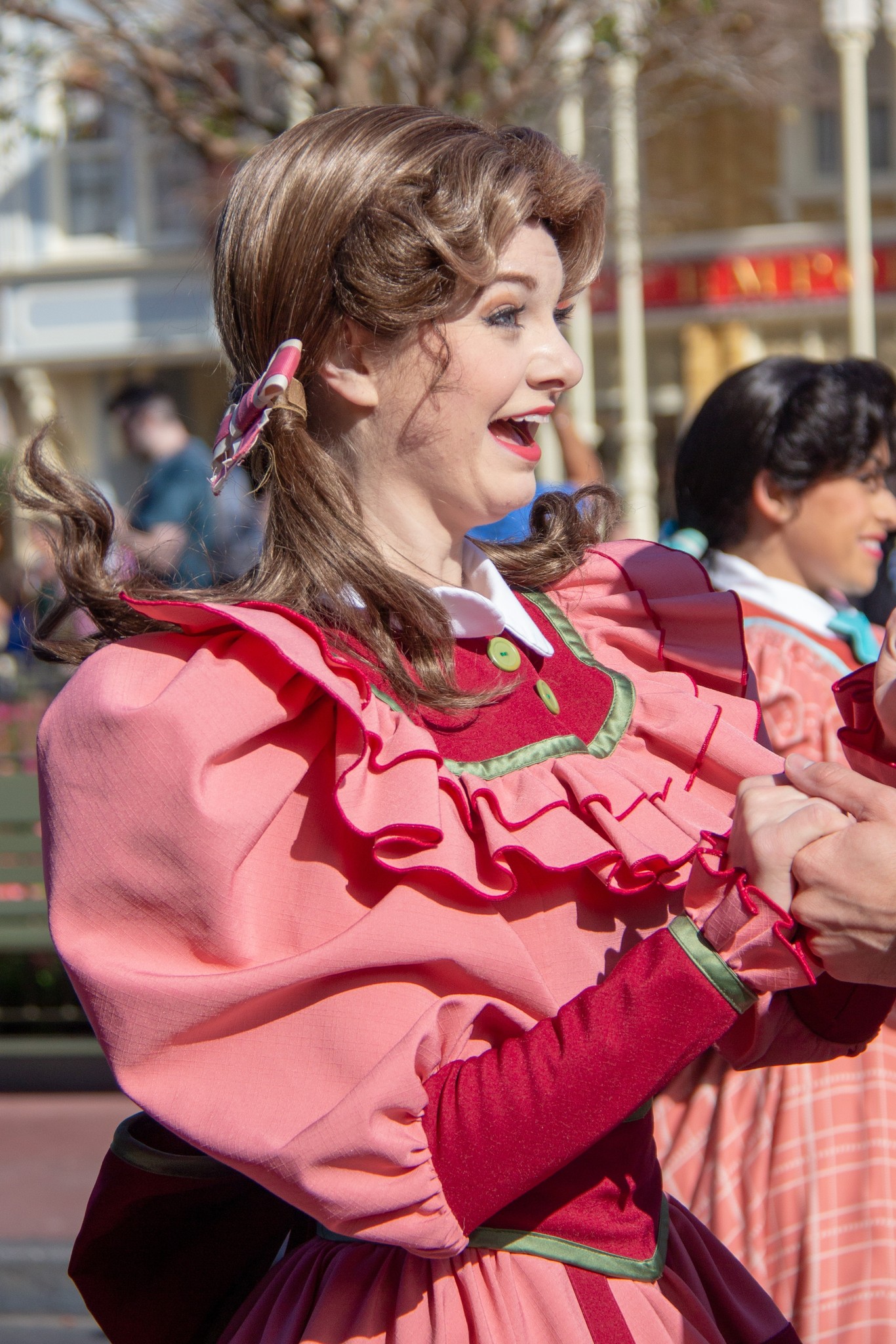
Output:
[67,1108,295,1344]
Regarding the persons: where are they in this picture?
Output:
[35,110,864,1344]
[662,355,895,1343]
[462,398,604,543]
[107,388,226,589]
[787,747,895,992]
